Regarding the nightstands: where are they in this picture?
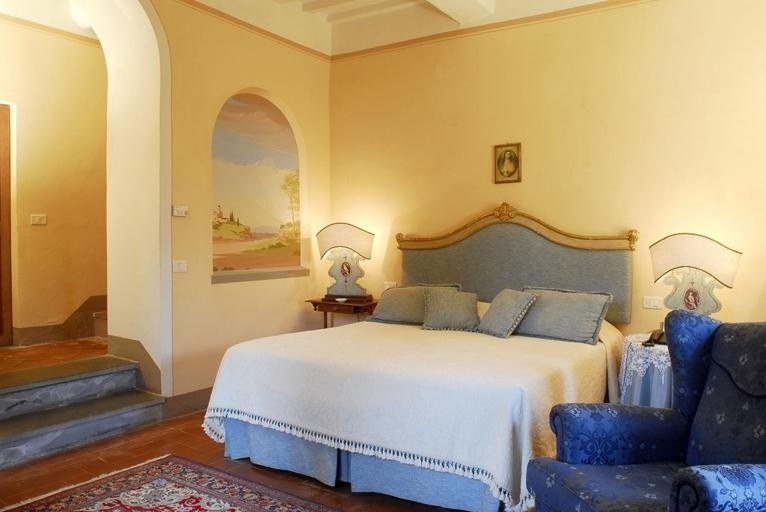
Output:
[620,332,674,408]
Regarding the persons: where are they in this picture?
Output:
[498,150,518,177]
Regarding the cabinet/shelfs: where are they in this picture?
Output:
[305,297,375,329]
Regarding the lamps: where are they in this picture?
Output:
[316,221,375,305]
[641,228,743,347]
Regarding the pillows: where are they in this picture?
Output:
[479,288,538,339]
[514,287,614,345]
[365,283,461,325]
[424,287,480,331]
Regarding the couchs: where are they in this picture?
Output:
[526,310,765,511]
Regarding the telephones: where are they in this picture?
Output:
[642,330,668,346]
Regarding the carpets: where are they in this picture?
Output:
[0,452,338,510]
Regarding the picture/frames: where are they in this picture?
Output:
[493,143,522,184]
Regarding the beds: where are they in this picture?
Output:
[202,202,640,511]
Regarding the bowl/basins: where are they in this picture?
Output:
[336,298,348,302]
